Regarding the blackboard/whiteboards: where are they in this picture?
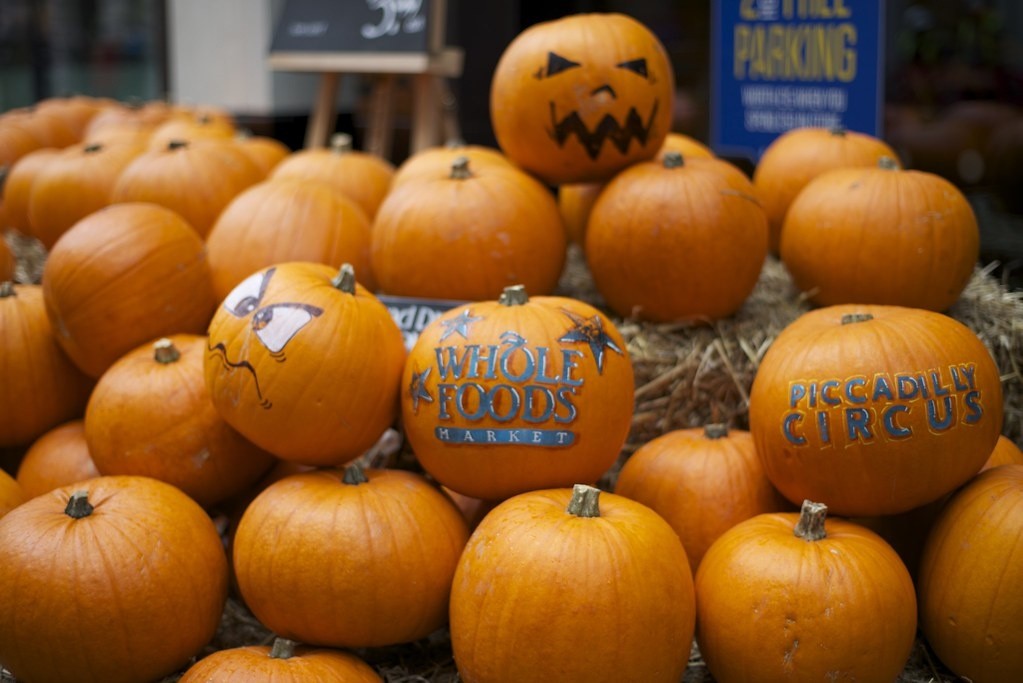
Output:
[268,0,429,56]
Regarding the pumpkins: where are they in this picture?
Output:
[0,99,1023,683]
